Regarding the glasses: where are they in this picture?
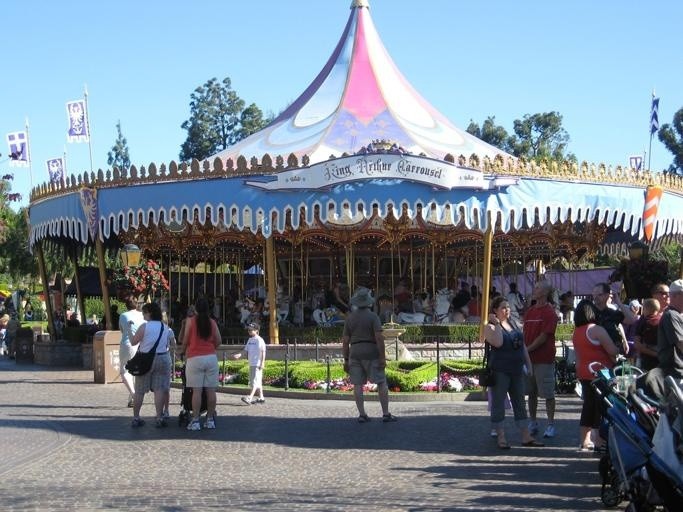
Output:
[655,291,670,296]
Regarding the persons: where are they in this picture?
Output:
[233,322,267,405]
[485,282,574,448]
[393,280,481,323]
[343,288,398,422]
[574,281,683,451]
[0,295,222,431]
[328,289,352,317]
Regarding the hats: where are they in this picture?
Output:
[347,286,377,308]
[629,299,644,308]
[668,279,683,293]
[245,323,259,331]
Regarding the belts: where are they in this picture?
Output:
[349,340,377,346]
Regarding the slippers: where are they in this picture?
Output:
[496,439,511,449]
[521,439,546,447]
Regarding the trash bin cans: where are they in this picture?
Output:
[93,330,123,384]
[16,327,34,364]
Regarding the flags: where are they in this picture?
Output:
[652,98,659,133]
[6,130,29,165]
[66,99,88,143]
[47,159,63,183]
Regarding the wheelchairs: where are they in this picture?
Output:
[554,346,577,394]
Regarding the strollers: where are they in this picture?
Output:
[588,359,683,511]
[177,350,218,428]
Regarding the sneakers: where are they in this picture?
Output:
[250,397,265,404]
[130,416,145,428]
[382,413,397,422]
[594,442,610,455]
[126,392,135,408]
[577,442,594,452]
[201,420,216,429]
[185,421,201,431]
[526,421,540,435]
[154,417,169,429]
[358,413,371,422]
[489,429,498,436]
[542,423,556,437]
[239,396,251,405]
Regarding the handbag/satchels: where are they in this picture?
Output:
[124,349,157,376]
[477,367,505,387]
